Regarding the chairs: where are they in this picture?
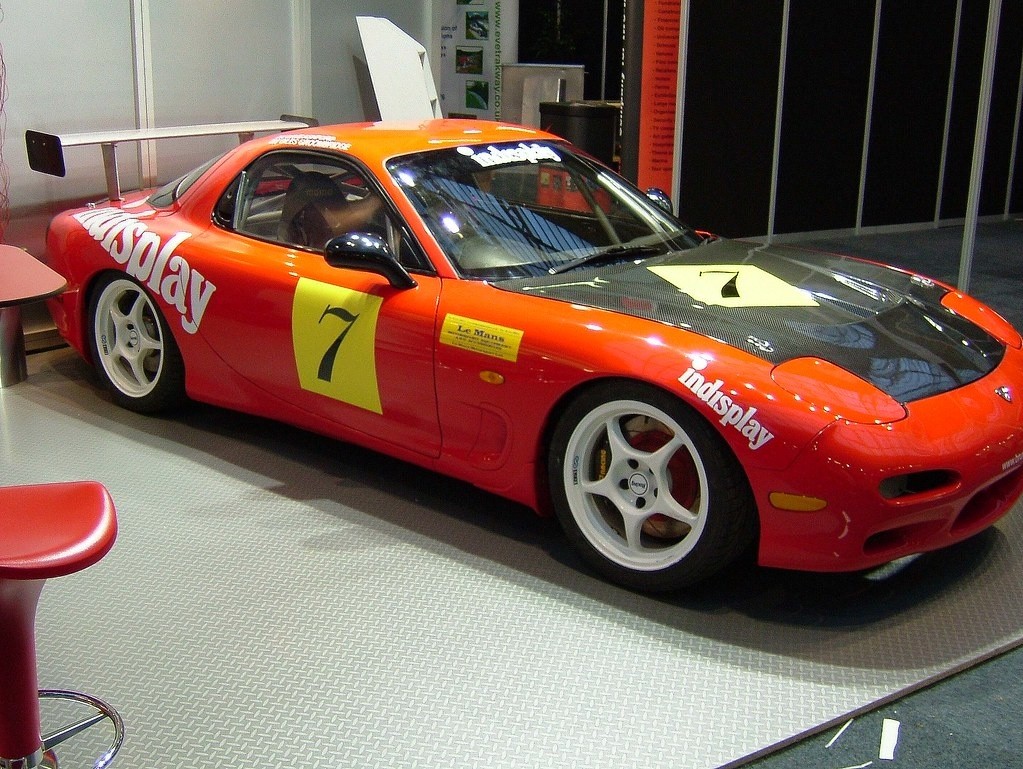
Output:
[281,170,387,252]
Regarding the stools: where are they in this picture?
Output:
[0,481,126,769]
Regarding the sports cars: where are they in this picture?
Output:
[24,116,1023,599]
